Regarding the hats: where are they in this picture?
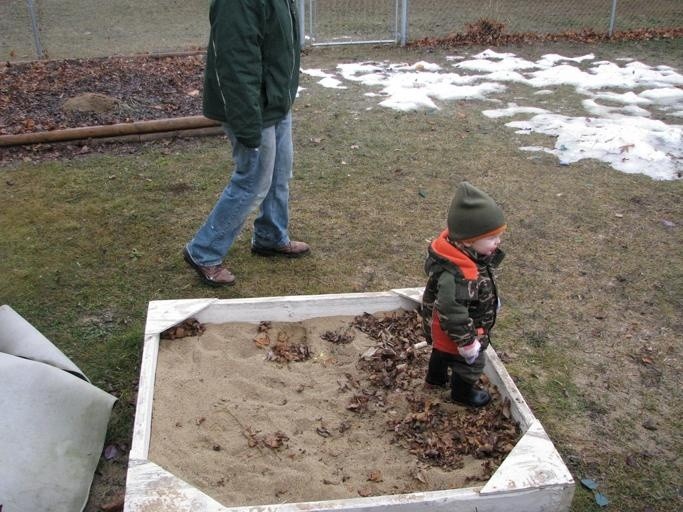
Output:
[447,181,507,242]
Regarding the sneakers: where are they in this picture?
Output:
[251,240,311,257]
[450,387,491,406]
[424,374,452,389]
[183,245,236,288]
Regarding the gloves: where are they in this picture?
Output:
[458,339,482,365]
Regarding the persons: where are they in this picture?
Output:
[421,180,507,408]
[183,0,311,284]
